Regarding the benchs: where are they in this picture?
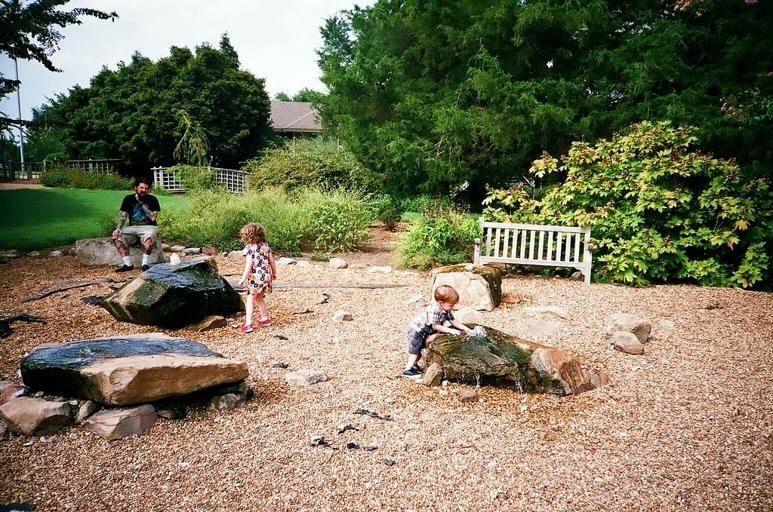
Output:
[472,216,594,286]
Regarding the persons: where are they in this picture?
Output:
[401,284,472,378]
[111,177,160,272]
[236,222,276,334]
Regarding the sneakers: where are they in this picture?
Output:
[400,369,418,375]
[412,363,422,374]
[240,324,253,332]
[256,320,271,327]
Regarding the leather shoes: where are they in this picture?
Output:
[115,263,133,272]
[141,265,149,271]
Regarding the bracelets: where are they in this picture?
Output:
[139,202,145,206]
